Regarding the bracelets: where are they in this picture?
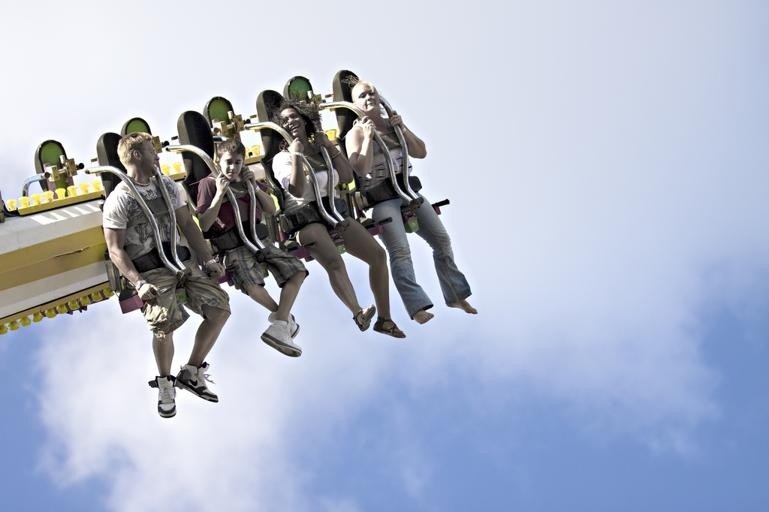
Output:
[326,144,341,159]
[136,279,146,291]
[401,125,408,135]
[206,258,216,266]
[290,151,304,158]
[254,185,260,193]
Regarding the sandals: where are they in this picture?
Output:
[372,315,407,340]
[351,304,379,334]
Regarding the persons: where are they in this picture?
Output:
[196,138,310,358]
[269,100,406,341]
[343,82,478,324]
[101,131,233,421]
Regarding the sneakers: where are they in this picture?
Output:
[154,375,177,419]
[261,324,304,359]
[173,360,222,404]
[267,311,300,342]
